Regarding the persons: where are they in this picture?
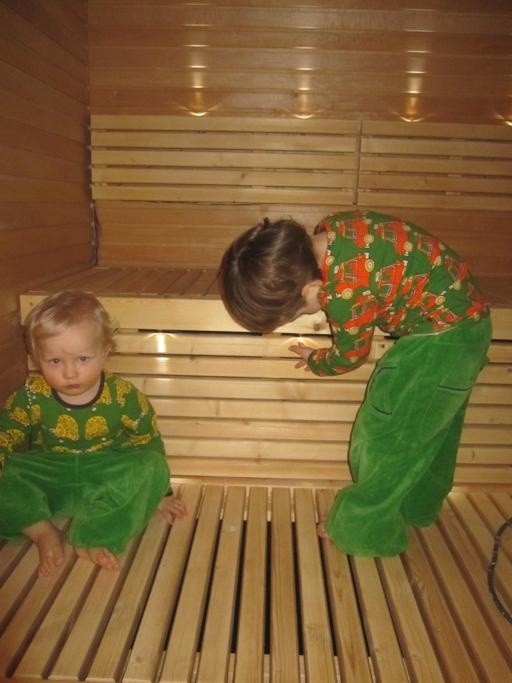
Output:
[218,211,492,558]
[1,289,187,576]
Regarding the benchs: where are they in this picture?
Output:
[17,114,512,485]
